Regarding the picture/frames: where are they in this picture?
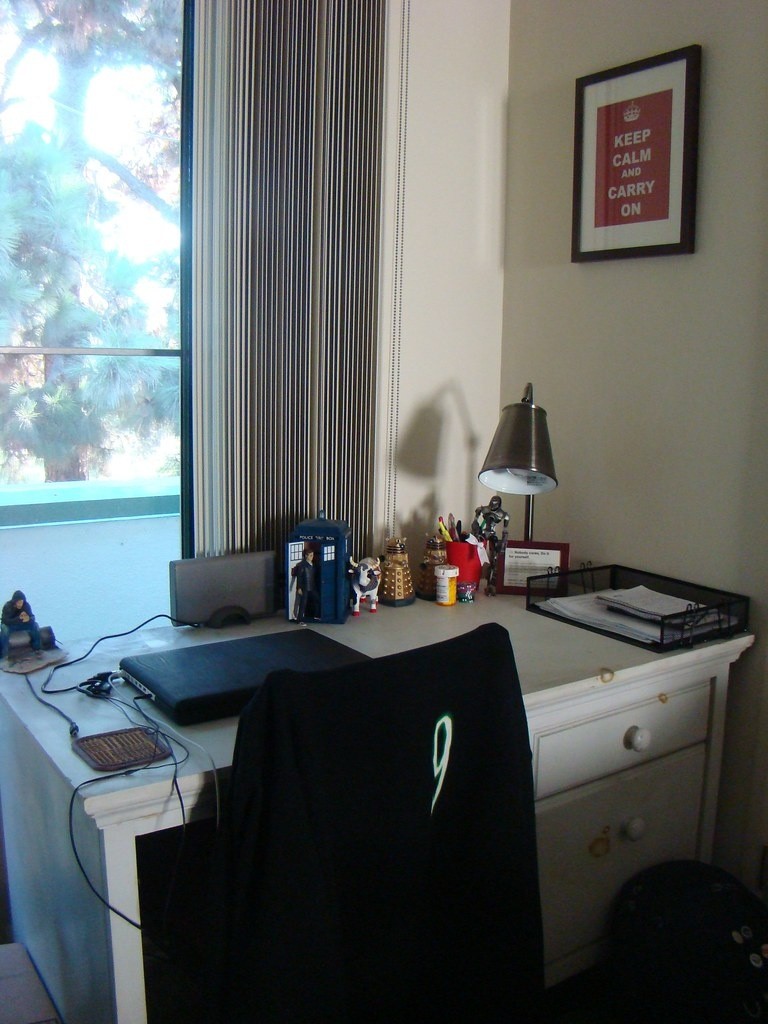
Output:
[496,539,570,599]
[570,44,701,262]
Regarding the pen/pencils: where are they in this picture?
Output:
[438,513,462,542]
[481,520,485,530]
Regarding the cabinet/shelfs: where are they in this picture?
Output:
[0,589,753,1024]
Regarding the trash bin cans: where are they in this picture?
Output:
[609,859,768,1024]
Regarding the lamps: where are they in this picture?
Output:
[477,382,558,541]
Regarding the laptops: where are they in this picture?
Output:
[116,626,374,726]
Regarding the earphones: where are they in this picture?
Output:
[98,681,112,694]
[86,686,104,697]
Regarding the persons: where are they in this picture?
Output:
[294,550,319,627]
[472,496,510,597]
[0,590,44,660]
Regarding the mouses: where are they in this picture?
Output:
[203,604,251,628]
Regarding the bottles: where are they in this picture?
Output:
[435,564,460,606]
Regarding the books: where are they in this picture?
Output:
[595,585,723,630]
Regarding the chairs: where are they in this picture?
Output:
[139,624,545,1024]
